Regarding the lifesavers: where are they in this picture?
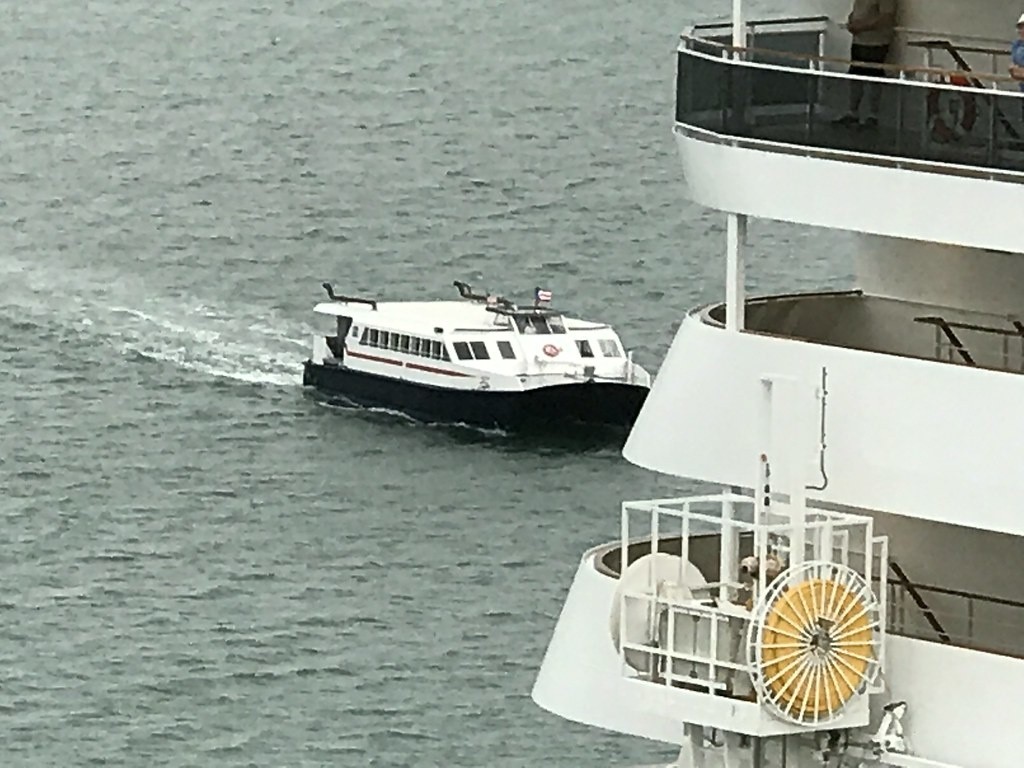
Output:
[923,71,980,145]
[543,343,560,357]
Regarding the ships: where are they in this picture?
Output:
[521,2,1024,767]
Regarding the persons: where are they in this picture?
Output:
[1008,10,1024,92]
[522,316,537,334]
[832,0,899,129]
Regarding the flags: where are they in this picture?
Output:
[535,288,552,302]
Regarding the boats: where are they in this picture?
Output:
[301,280,655,426]
